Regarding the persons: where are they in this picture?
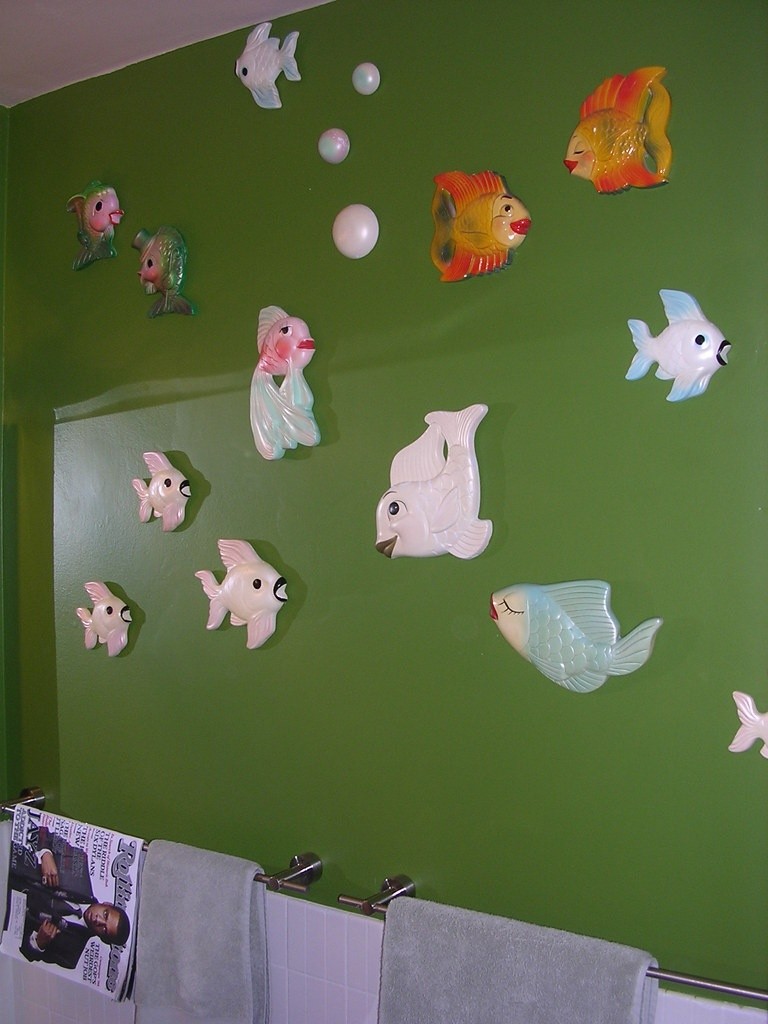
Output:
[3,826,130,969]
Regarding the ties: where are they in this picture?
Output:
[52,898,83,919]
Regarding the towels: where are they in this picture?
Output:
[376,895,660,1023]
[130,840,271,1024]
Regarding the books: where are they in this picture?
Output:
[0,803,148,1003]
